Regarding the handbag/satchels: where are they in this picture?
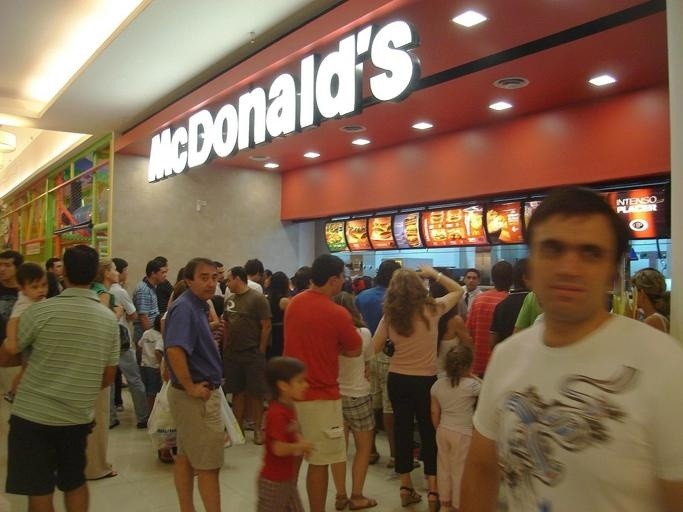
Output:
[383,339,395,357]
[97,290,131,351]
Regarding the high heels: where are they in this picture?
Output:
[399,486,422,506]
[426,492,441,512]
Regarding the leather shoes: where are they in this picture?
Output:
[109,420,120,430]
[136,421,146,428]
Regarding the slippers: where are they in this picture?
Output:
[158,449,174,464]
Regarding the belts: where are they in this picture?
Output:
[171,382,220,391]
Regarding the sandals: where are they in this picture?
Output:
[334,494,350,510]
[369,451,380,464]
[385,457,420,469]
[349,494,378,510]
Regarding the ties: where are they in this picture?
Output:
[465,292,469,307]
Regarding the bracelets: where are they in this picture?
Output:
[436,272,443,283]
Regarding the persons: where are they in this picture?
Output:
[290,266,315,297]
[132,259,166,365]
[46,257,64,276]
[269,272,289,357]
[83,255,118,481]
[458,186,683,512]
[212,260,225,296]
[90,260,124,428]
[283,255,363,511]
[136,313,164,410]
[333,291,378,511]
[262,270,272,294]
[629,270,671,334]
[6,246,121,511]
[1,263,47,403]
[455,268,483,321]
[175,266,219,328]
[0,250,24,344]
[109,258,149,429]
[512,292,542,335]
[371,263,465,512]
[465,262,514,379]
[342,281,355,294]
[363,276,374,289]
[224,258,264,303]
[155,256,175,314]
[489,259,532,351]
[211,295,225,359]
[163,257,227,512]
[428,270,473,380]
[355,260,401,468]
[256,356,317,512]
[221,266,272,449]
[114,369,124,412]
[430,344,482,512]
[153,276,218,462]
